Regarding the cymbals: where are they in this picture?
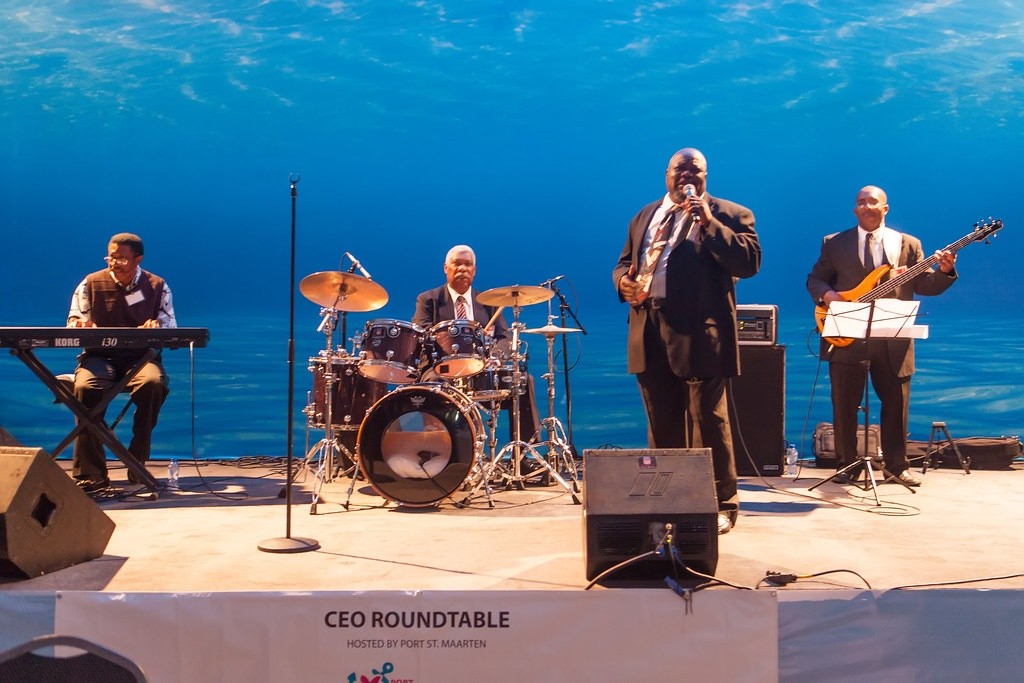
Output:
[298,271,390,313]
[520,324,584,337]
[475,285,555,308]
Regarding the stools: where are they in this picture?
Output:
[54,373,136,442]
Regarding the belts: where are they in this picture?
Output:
[643,296,667,310]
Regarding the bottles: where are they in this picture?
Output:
[167,458,180,491]
[786,444,799,477]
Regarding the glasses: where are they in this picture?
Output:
[104,256,138,266]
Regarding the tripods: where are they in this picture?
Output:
[461,284,588,511]
[278,288,359,515]
[808,299,929,506]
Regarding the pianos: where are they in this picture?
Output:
[0,327,210,502]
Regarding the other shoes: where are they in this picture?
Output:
[127,468,168,486]
[74,476,111,493]
[718,515,733,534]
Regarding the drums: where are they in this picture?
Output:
[425,318,486,379]
[357,318,428,385]
[307,355,389,431]
[356,381,486,506]
[467,364,528,401]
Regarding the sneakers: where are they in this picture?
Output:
[882,466,921,485]
[830,465,858,483]
[506,457,559,486]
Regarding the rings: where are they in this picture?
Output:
[942,260,947,263]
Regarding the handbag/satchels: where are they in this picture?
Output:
[812,420,881,464]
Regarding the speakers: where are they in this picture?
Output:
[722,343,788,474]
[0,447,114,581]
[580,448,719,584]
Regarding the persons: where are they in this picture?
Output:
[613,148,761,534]
[65,233,178,494]
[414,245,544,476]
[806,186,958,485]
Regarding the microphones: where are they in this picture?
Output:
[539,275,565,287]
[683,183,700,224]
[345,251,371,279]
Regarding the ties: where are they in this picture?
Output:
[633,204,683,306]
[864,233,877,289]
[457,295,467,319]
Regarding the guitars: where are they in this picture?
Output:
[814,216,1004,349]
